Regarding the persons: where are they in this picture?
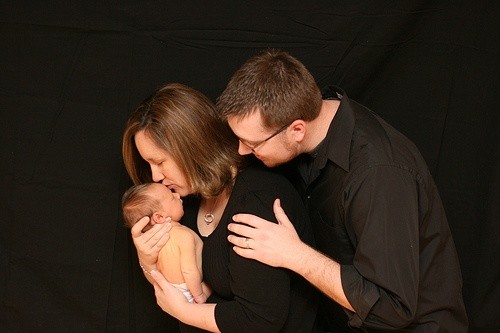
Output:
[122,183,227,333]
[215,51,469,333]
[123,84,323,333]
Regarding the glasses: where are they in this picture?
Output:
[241,124,290,153]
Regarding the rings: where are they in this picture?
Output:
[246,238,249,249]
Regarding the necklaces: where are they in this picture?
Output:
[203,165,233,225]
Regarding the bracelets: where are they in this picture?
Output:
[140,263,151,276]
